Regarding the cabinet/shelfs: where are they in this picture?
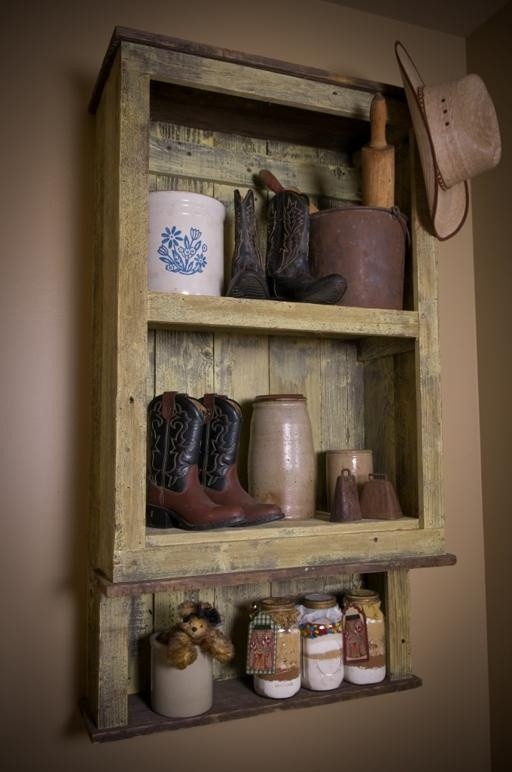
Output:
[65,24,461,750]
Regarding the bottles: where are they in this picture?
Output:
[326,449,374,513]
[150,191,227,296]
[246,395,318,520]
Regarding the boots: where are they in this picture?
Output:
[147,390,285,530]
[223,188,348,302]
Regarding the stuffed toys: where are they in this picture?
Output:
[159,598,236,670]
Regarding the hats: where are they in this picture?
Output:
[392,35,504,242]
